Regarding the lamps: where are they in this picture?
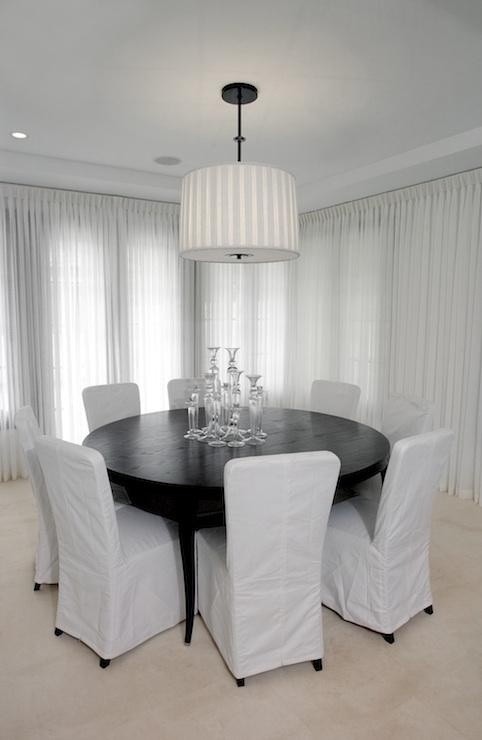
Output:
[179,82,300,264]
